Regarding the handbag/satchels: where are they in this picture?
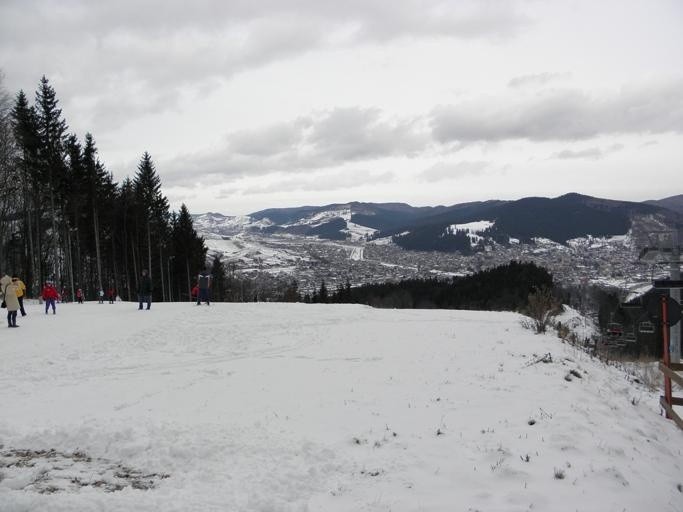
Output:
[1,302,7,308]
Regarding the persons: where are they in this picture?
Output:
[191,282,199,302]
[0,270,27,327]
[196,266,211,305]
[137,269,153,309]
[42,279,113,315]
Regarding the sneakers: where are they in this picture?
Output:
[7,324,18,327]
